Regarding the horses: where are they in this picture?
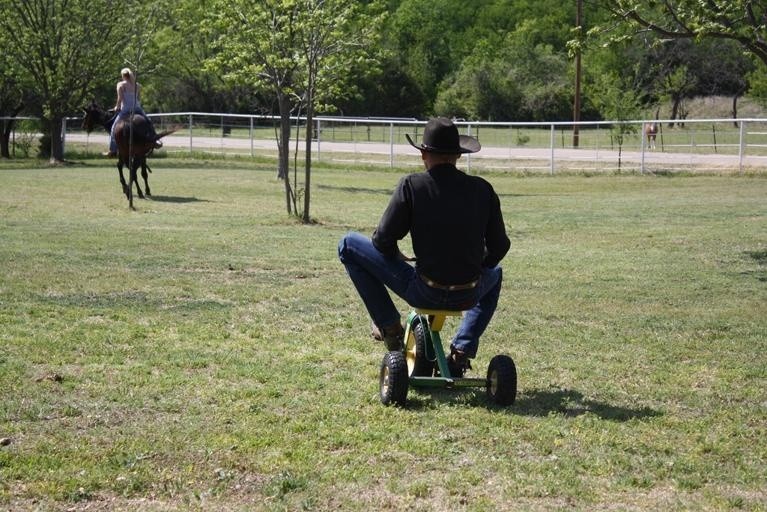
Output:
[81,97,181,200]
[645,122,659,151]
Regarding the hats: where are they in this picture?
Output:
[405,117,481,154]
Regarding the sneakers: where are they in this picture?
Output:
[449,341,470,365]
[373,321,402,349]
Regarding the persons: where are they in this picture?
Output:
[338,118,510,369]
[102,67,162,157]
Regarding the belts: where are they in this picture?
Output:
[419,274,479,292]
